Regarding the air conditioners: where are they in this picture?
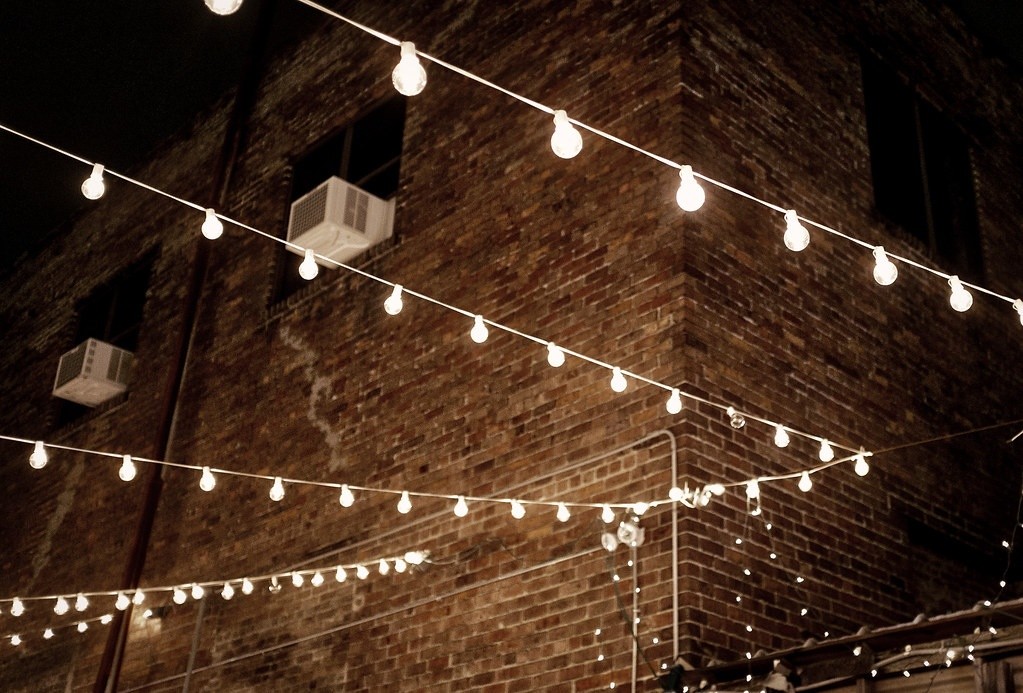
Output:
[285,177,396,266]
[53,337,134,409]
